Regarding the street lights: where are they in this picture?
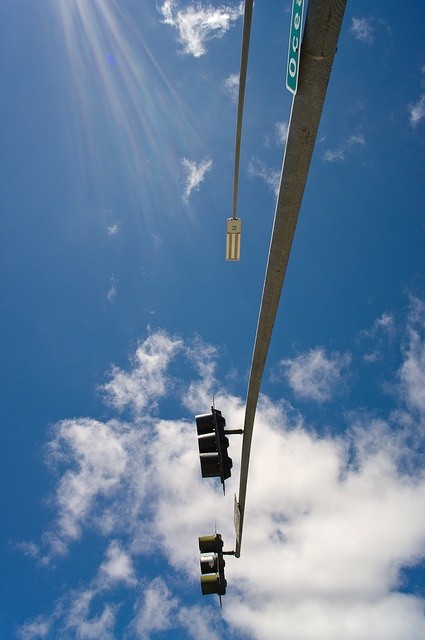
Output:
[226,4,254,258]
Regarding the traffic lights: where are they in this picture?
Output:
[195,397,232,495]
[197,519,228,611]
